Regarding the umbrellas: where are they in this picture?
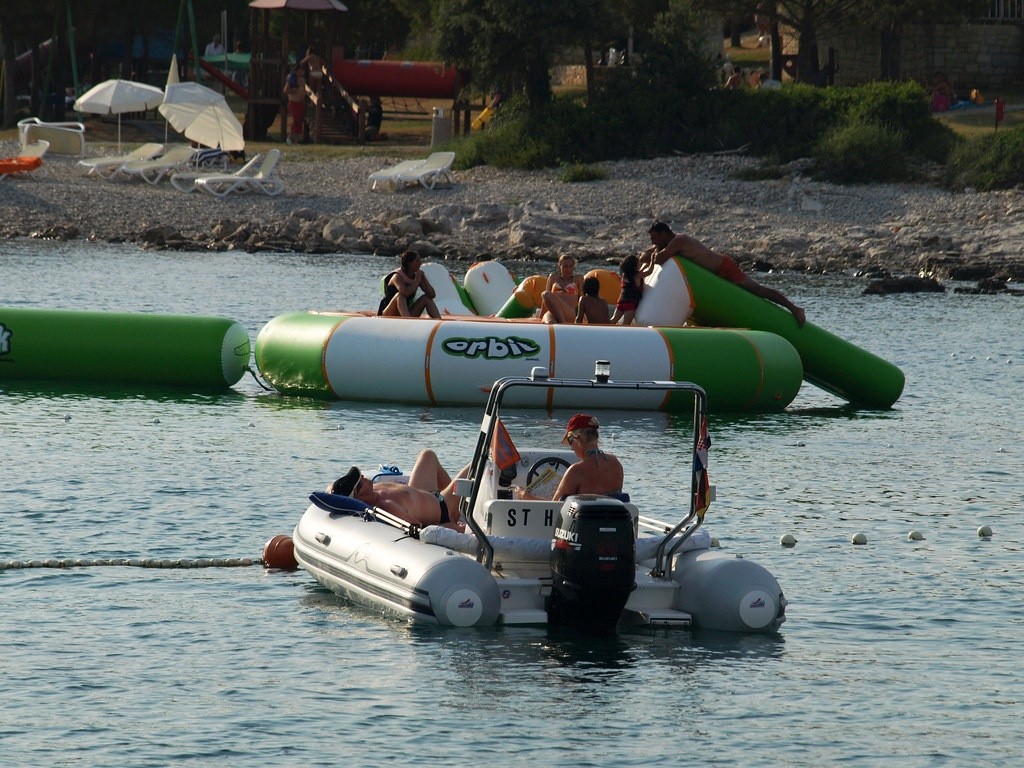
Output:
[159,82,244,171]
[73,79,164,147]
[165,54,179,142]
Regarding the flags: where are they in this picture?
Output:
[696,422,711,518]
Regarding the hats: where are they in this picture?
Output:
[561,414,598,445]
[331,466,361,496]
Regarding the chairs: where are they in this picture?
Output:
[80,143,164,182]
[121,145,195,187]
[0,138,52,183]
[195,148,286,200]
[16,117,87,158]
[170,152,260,198]
[368,150,457,193]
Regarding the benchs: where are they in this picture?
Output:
[486,496,638,566]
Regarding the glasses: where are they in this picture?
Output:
[568,437,573,445]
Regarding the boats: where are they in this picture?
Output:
[251,249,907,419]
[262,358,790,641]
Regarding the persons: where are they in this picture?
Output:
[331,451,473,532]
[724,67,780,90]
[488,83,507,111]
[377,251,440,318]
[516,414,623,502]
[610,251,656,325]
[574,276,611,324]
[299,46,325,98]
[204,35,245,87]
[928,72,956,113]
[639,222,805,325]
[539,255,583,324]
[284,65,310,146]
